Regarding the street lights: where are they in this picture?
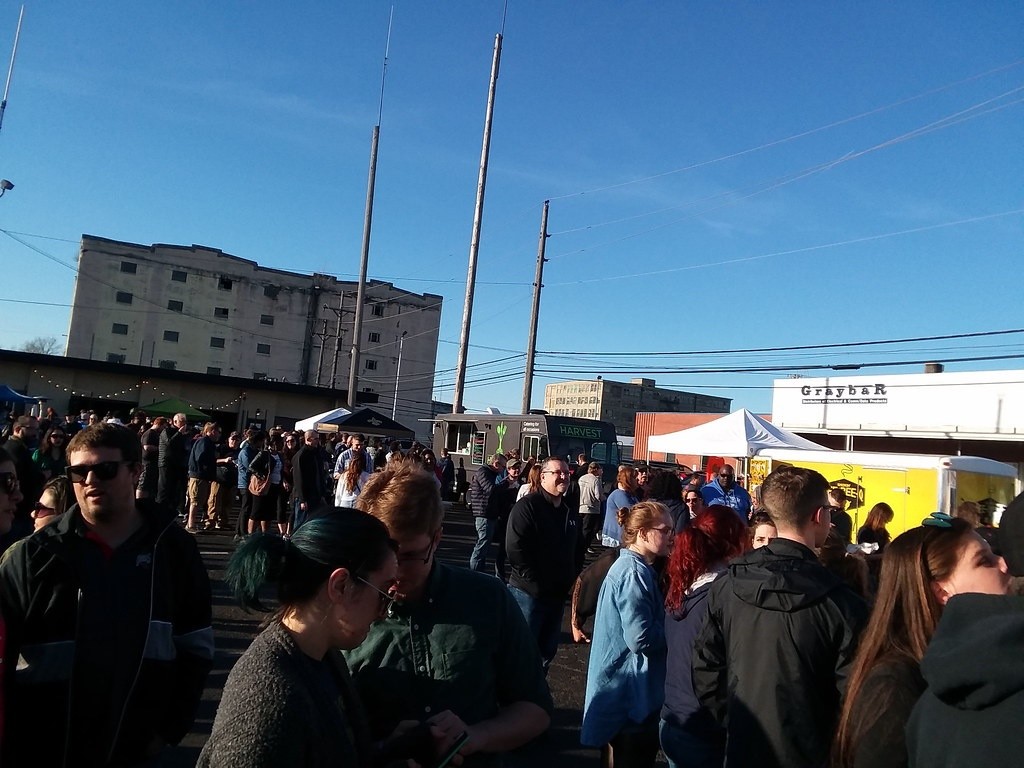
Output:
[595,375,602,422]
[392,331,408,420]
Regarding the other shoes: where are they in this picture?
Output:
[185,521,232,534]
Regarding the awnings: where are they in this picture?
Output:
[417,418,478,449]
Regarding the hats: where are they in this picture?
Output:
[506,459,521,469]
[633,460,650,469]
[64,409,76,415]
[231,431,241,438]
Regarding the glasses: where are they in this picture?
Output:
[499,463,506,469]
[350,570,398,616]
[0,472,19,494]
[22,426,34,430]
[35,502,60,518]
[719,472,733,478]
[51,434,66,439]
[923,513,955,584]
[811,505,839,521]
[286,439,295,443]
[399,530,437,567]
[685,498,701,502]
[64,461,133,483]
[543,471,571,478]
[650,525,675,537]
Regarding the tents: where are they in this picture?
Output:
[130,396,210,425]
[645,407,831,490]
[295,407,351,431]
[319,407,416,441]
[0,385,54,404]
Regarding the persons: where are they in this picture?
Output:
[0,406,1024,768]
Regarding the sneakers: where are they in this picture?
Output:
[587,547,594,553]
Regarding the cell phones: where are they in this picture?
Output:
[436,730,469,768]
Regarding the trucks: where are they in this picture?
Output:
[418,407,625,511]
[751,447,1018,555]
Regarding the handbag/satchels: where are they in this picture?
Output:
[248,451,271,496]
